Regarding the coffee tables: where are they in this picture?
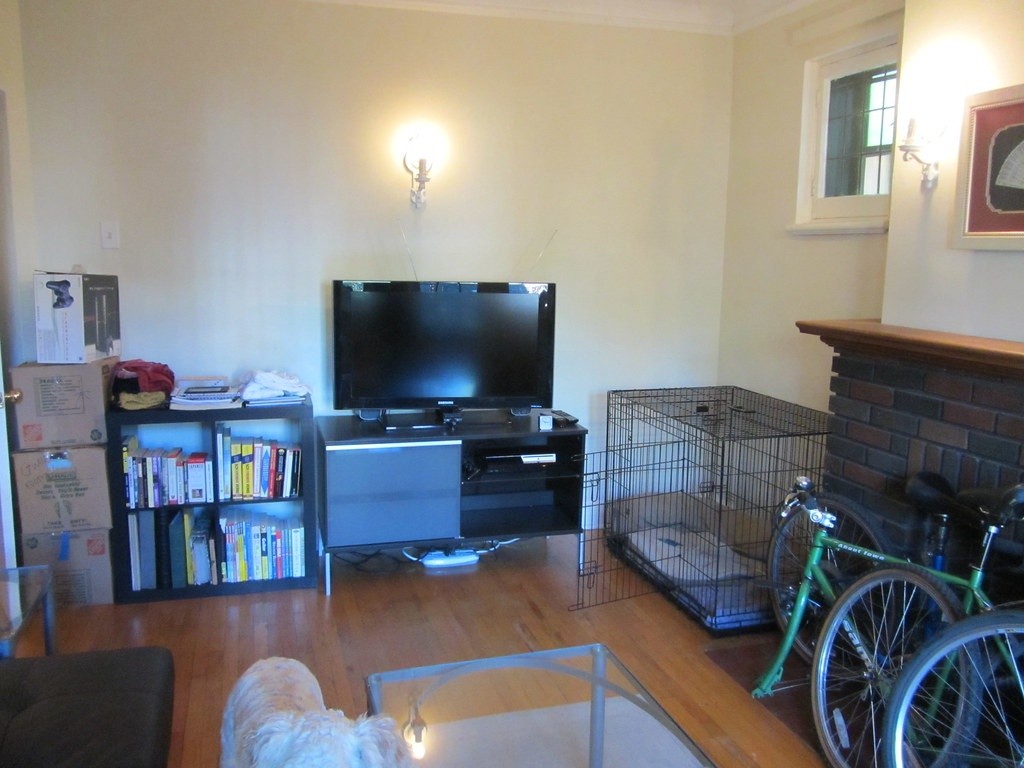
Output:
[365,640,714,768]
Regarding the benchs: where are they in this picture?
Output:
[0,645,178,768]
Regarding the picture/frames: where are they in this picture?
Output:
[946,81,1024,253]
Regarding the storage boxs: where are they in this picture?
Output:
[8,356,121,451]
[20,529,121,606]
[9,446,114,535]
[32,268,122,363]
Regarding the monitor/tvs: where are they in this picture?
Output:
[333,279,556,429]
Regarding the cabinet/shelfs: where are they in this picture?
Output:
[314,408,588,595]
[105,392,319,603]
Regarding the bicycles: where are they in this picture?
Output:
[751,471,1024,768]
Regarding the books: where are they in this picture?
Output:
[170,375,243,409]
[122,423,306,591]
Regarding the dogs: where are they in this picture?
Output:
[216,653,413,768]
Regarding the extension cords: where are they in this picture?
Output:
[421,549,479,569]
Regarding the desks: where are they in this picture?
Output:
[0,566,55,656]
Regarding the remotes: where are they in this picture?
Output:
[539,409,579,431]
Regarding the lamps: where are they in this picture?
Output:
[386,118,451,208]
[897,99,945,192]
[400,677,430,761]
